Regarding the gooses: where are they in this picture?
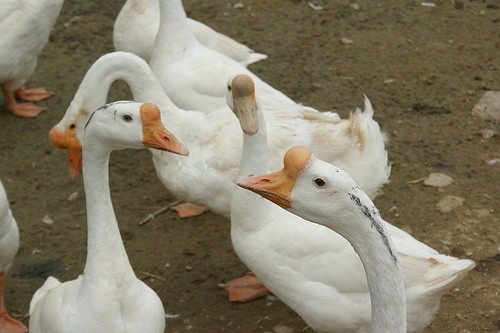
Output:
[221,75,477,333]
[0,0,266,333]
